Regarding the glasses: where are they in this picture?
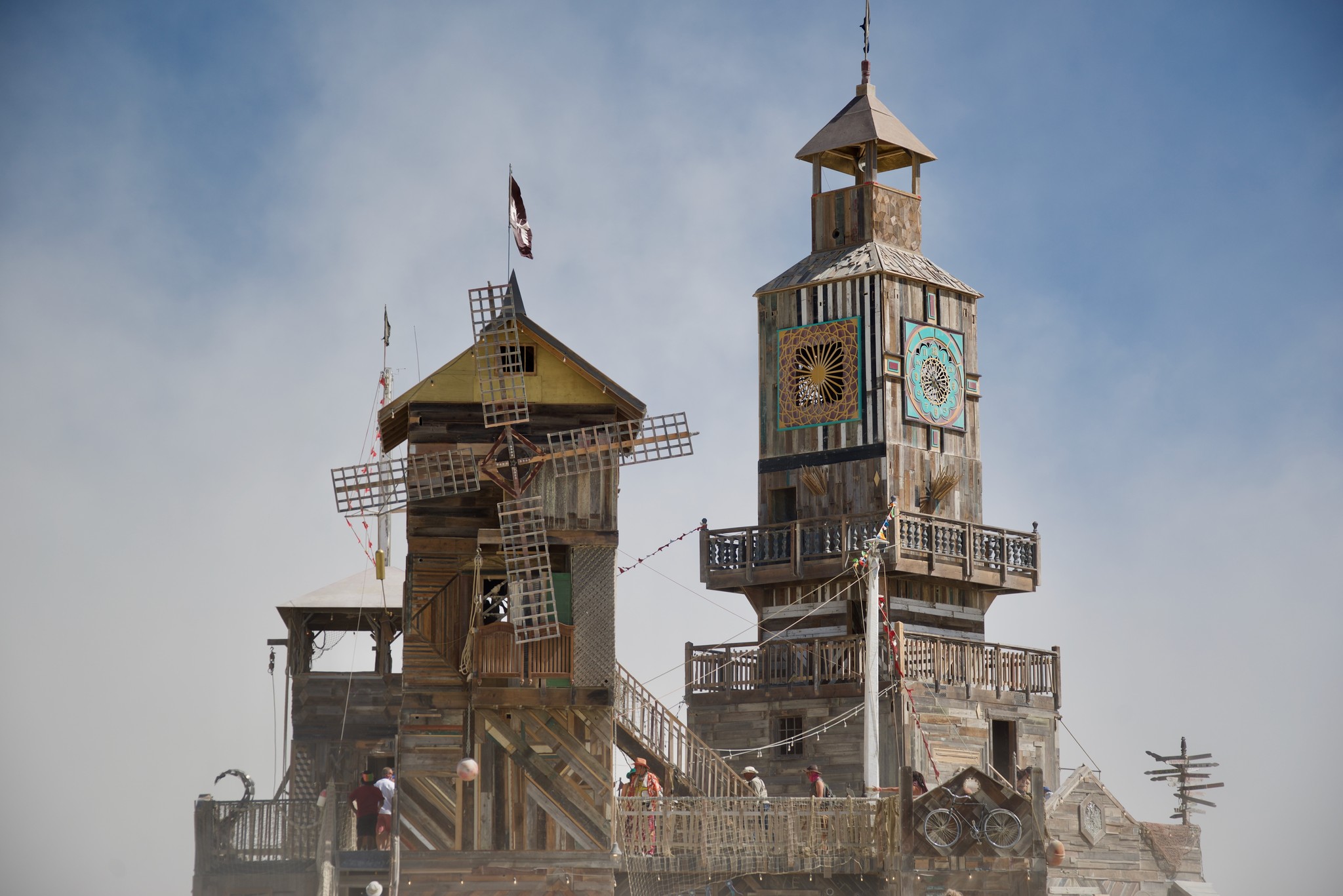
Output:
[384,772,394,776]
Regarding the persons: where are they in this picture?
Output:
[374,767,395,851]
[872,771,928,799]
[943,889,963,896]
[802,764,832,855]
[347,770,385,851]
[1017,766,1033,794]
[741,766,775,851]
[366,881,383,896]
[1043,787,1054,801]
[621,757,661,857]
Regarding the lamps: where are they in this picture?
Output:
[858,156,866,173]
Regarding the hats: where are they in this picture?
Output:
[627,769,636,778]
[362,770,374,782]
[1025,766,1033,778]
[802,765,822,774]
[1043,787,1053,792]
[740,766,758,774]
[366,881,383,896]
[630,757,650,769]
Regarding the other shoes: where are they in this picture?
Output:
[628,853,652,858]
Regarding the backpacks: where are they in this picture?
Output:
[813,778,835,809]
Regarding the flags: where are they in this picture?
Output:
[346,376,387,568]
[853,500,898,567]
[509,175,534,259]
[381,307,391,346]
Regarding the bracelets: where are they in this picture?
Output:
[879,788,881,791]
[1022,778,1024,781]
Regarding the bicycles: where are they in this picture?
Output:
[924,785,1023,848]
